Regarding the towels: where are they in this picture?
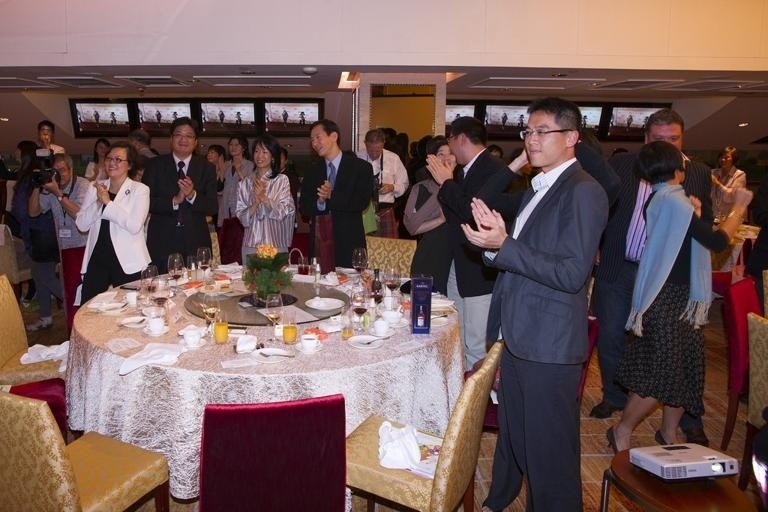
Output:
[119,342,182,376]
[20,339,69,364]
[378,421,421,470]
[86,291,118,308]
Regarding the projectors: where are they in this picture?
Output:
[628,442,739,483]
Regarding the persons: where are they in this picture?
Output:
[461,96,610,512]
[591,108,712,448]
[28,153,90,341]
[456,114,460,119]
[426,117,514,371]
[235,112,242,128]
[298,119,373,275]
[206,144,226,234]
[626,115,632,132]
[128,129,156,157]
[583,116,587,127]
[468,138,622,512]
[38,120,66,154]
[2,141,39,309]
[298,112,306,128]
[156,110,162,128]
[606,142,753,455]
[141,116,219,275]
[222,135,254,219]
[502,113,507,129]
[75,140,153,307]
[356,130,410,210]
[280,147,298,207]
[0,158,22,179]
[25,150,64,331]
[85,137,112,179]
[281,110,288,128]
[173,112,177,120]
[488,144,503,156]
[580,131,629,159]
[746,172,768,278]
[93,111,100,128]
[642,116,648,133]
[404,135,456,298]
[236,137,295,267]
[382,128,431,172]
[110,112,118,128]
[518,115,524,128]
[218,110,225,127]
[711,146,747,223]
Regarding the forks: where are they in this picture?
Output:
[259,351,295,359]
[359,336,390,345]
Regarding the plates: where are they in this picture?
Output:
[431,315,449,327]
[295,343,323,354]
[348,335,384,349]
[180,339,206,350]
[252,348,288,364]
[143,327,169,336]
[305,298,345,310]
[122,317,147,328]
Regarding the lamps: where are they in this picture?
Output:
[303,66,318,75]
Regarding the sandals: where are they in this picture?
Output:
[26,317,53,331]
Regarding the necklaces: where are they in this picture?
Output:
[253,171,273,222]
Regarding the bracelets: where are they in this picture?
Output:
[728,208,745,224]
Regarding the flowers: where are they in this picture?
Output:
[242,242,293,295]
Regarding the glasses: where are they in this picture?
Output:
[105,156,128,162]
[520,126,573,140]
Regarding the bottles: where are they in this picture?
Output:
[412,276,433,334]
[197,262,202,281]
[191,263,196,281]
[312,257,317,274]
[315,263,321,282]
[369,299,376,322]
[372,269,381,292]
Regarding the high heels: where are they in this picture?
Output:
[606,425,619,455]
[654,430,667,446]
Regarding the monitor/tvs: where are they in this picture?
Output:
[605,101,674,143]
[197,96,260,139]
[571,101,604,143]
[482,98,533,142]
[444,99,481,133]
[67,98,133,138]
[134,98,196,138]
[260,98,326,139]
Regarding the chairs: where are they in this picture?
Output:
[712,271,731,297]
[1,391,171,511]
[345,343,495,512]
[1,378,68,442]
[315,214,335,275]
[357,235,415,282]
[761,270,766,317]
[743,314,768,463]
[0,222,35,284]
[721,275,764,450]
[61,246,90,333]
[208,224,222,265]
[0,274,69,385]
[200,394,348,511]
[220,217,245,263]
[290,224,309,263]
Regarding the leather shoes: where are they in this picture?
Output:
[680,413,709,446]
[590,400,624,418]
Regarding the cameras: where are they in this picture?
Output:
[372,174,383,199]
[30,149,61,189]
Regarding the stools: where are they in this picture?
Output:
[601,448,755,512]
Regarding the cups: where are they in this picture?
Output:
[266,294,283,345]
[386,268,402,311]
[202,293,220,338]
[183,329,201,346]
[301,335,321,351]
[168,254,184,292]
[351,247,368,273]
[141,265,160,307]
[150,278,170,320]
[283,310,298,346]
[351,286,366,333]
[214,310,228,344]
[374,320,388,336]
[147,318,164,332]
[298,257,309,275]
[340,306,354,340]
[196,247,212,282]
[382,311,404,325]
[124,293,137,307]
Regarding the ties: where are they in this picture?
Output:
[176,161,186,225]
[328,163,336,190]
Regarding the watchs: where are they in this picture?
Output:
[57,195,68,201]
